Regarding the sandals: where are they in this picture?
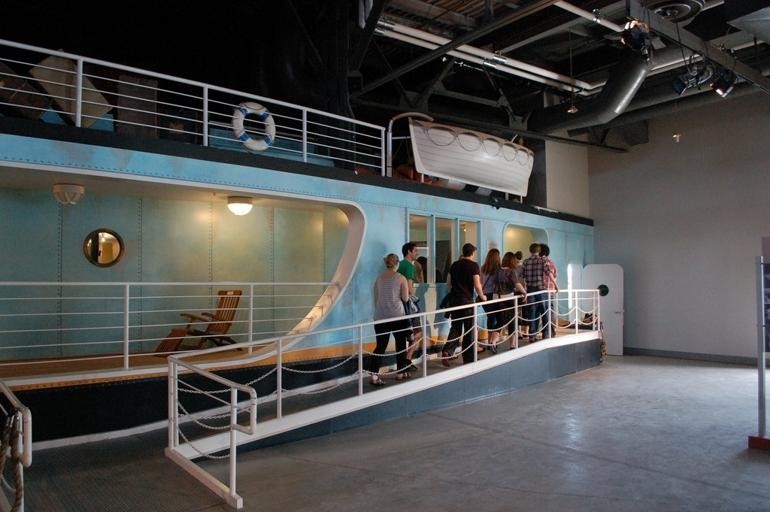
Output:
[369,377,389,386]
[476,346,487,354]
[395,371,412,381]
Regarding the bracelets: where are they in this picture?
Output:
[418,268,423,272]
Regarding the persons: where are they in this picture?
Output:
[396,243,424,373]
[369,253,412,386]
[417,256,441,284]
[442,242,487,366]
[478,242,558,354]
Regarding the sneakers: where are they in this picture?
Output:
[509,346,515,350]
[486,342,498,354]
[404,358,418,371]
[441,351,450,368]
[517,331,556,344]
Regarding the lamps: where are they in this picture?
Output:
[52,183,85,206]
[225,196,254,215]
[622,1,770,102]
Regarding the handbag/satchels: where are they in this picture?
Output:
[475,293,482,308]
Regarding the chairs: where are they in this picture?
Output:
[154,291,244,354]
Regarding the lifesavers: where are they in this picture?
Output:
[233,102,275,151]
